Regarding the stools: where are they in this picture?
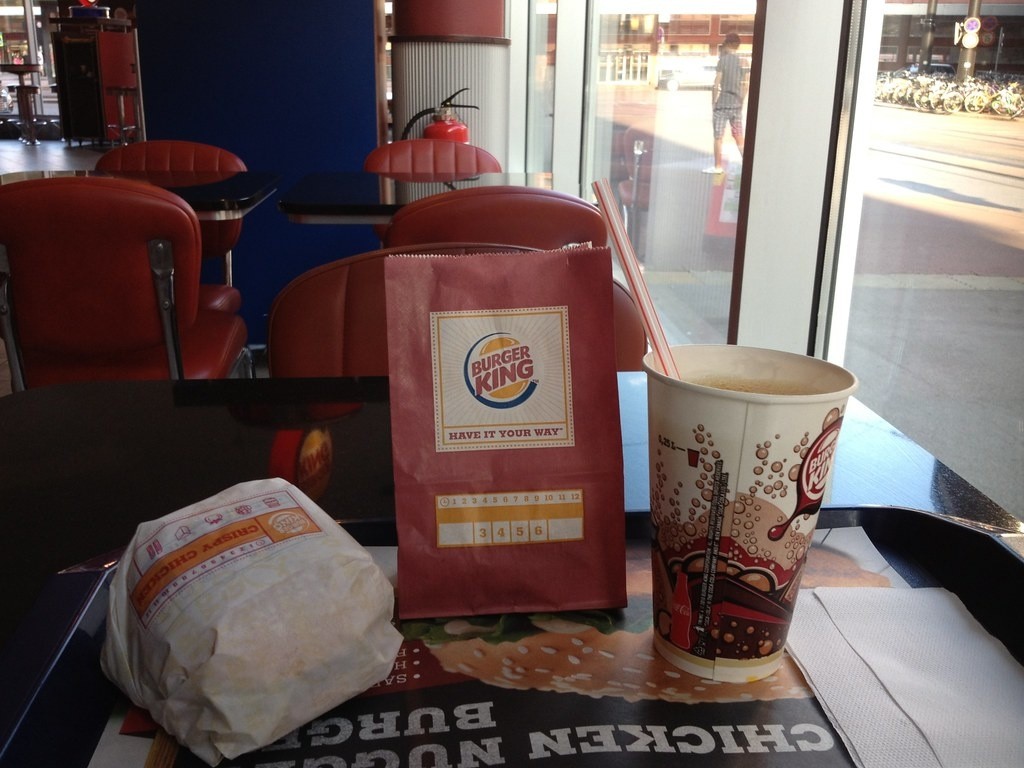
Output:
[7,85,41,146]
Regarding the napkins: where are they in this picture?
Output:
[782,588,1024,768]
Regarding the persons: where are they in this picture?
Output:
[657,22,664,54]
[38,43,56,77]
[702,32,750,174]
[13,52,25,65]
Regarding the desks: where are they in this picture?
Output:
[1,375,1024,768]
[282,170,598,224]
[0,171,278,289]
[0,64,42,145]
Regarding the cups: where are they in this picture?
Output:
[644,344,859,682]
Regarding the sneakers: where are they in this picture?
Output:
[702,166,723,174]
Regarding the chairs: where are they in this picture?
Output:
[0,138,648,374]
